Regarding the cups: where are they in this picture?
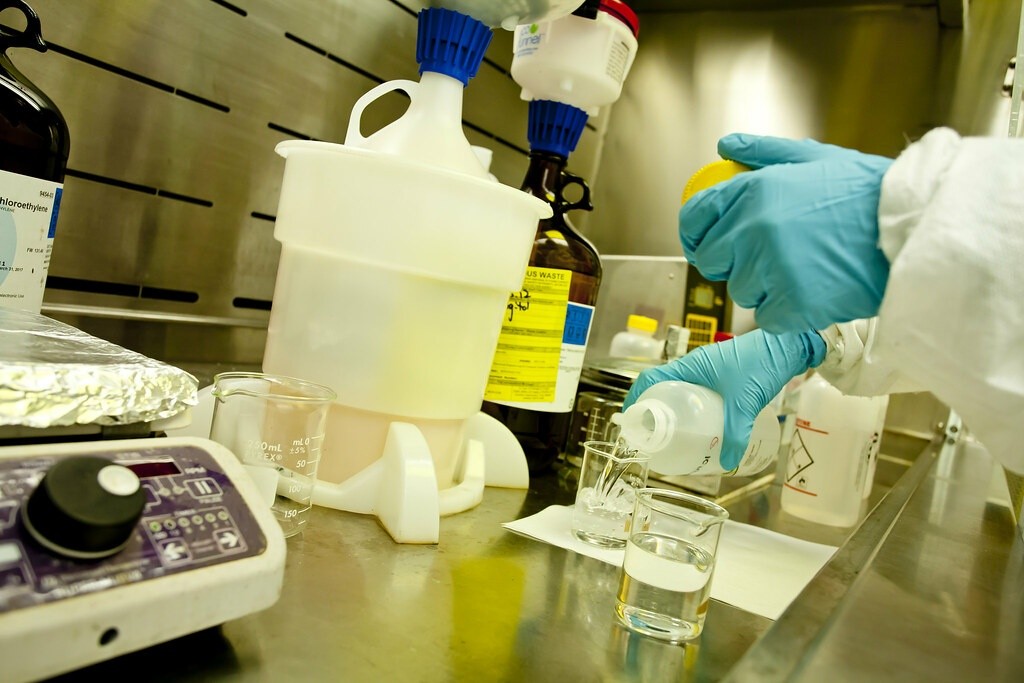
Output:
[573,440,652,548]
[615,487,729,641]
[208,371,336,537]
[565,393,622,469]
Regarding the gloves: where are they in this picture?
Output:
[622,329,828,470]
[677,133,893,334]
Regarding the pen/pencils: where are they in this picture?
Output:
[714,473,775,505]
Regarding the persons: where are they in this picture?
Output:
[615,126,1024,475]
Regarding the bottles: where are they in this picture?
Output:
[781,371,889,528]
[611,380,781,477]
[0,1,70,315]
[480,150,602,471]
[610,315,662,360]
[260,70,553,489]
